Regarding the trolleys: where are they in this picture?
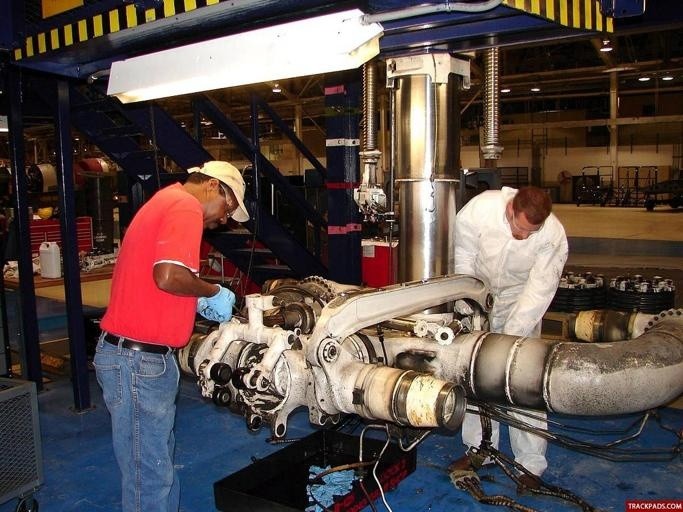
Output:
[576,165,659,207]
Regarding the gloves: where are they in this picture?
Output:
[196,283,236,324]
[304,465,368,511]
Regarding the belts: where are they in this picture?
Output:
[101,331,179,354]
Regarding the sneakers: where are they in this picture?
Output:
[446,452,498,476]
[516,474,541,494]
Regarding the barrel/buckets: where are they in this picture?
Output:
[607,282,675,313]
[39,242,61,279]
[546,285,608,312]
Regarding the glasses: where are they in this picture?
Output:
[511,211,545,235]
[209,178,235,219]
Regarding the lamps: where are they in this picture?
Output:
[107,7,384,105]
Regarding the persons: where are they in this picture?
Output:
[92,160,249,512]
[453,185,569,497]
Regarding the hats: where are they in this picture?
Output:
[186,160,249,223]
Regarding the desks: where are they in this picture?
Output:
[2,264,118,367]
